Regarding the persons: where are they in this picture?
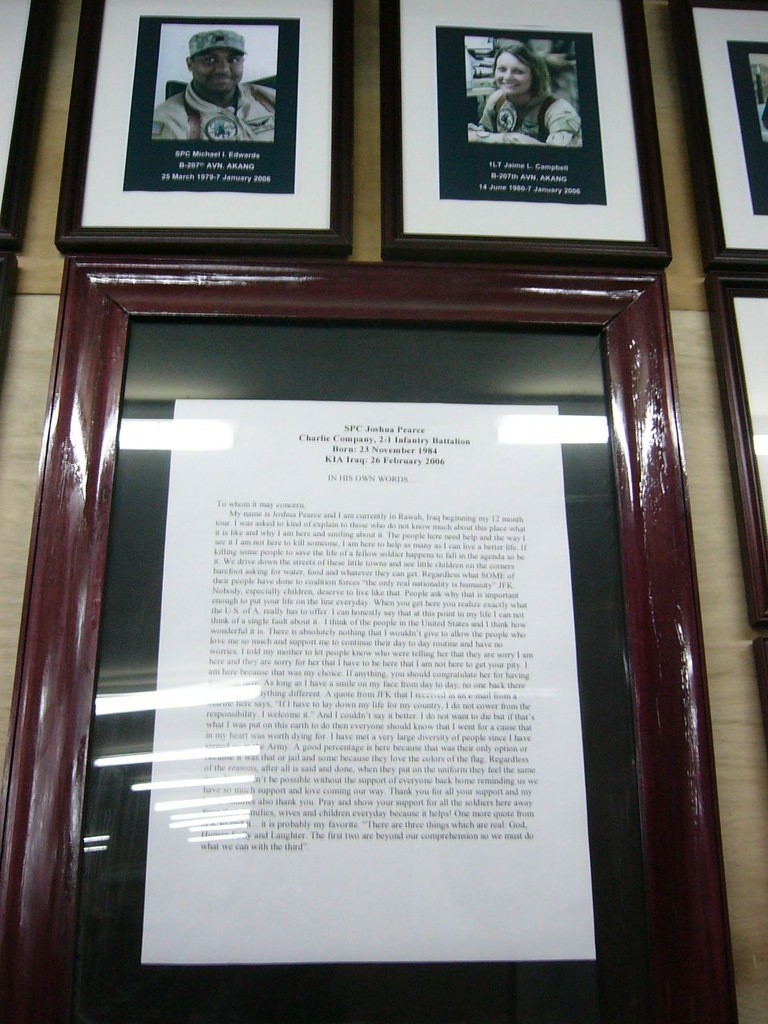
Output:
[478,42,582,147]
[151,30,276,141]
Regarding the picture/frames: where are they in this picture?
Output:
[703,274,768,629]
[670,1,767,277]
[0,1,58,248]
[55,1,359,255]
[378,0,669,270]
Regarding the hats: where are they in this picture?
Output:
[188,30,249,58]
